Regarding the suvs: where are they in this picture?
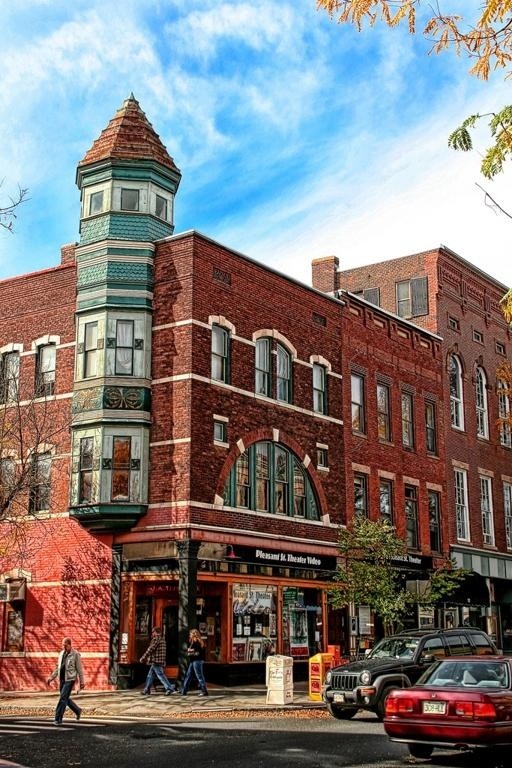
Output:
[320,627,498,723]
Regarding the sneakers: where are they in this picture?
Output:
[52,721,61,726]
[164,691,173,695]
[76,708,82,719]
[198,692,208,696]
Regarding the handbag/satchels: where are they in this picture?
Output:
[186,647,199,656]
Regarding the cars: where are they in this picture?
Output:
[383,654,511,759]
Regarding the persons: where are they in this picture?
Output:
[47,637,85,726]
[140,627,174,696]
[181,629,208,696]
